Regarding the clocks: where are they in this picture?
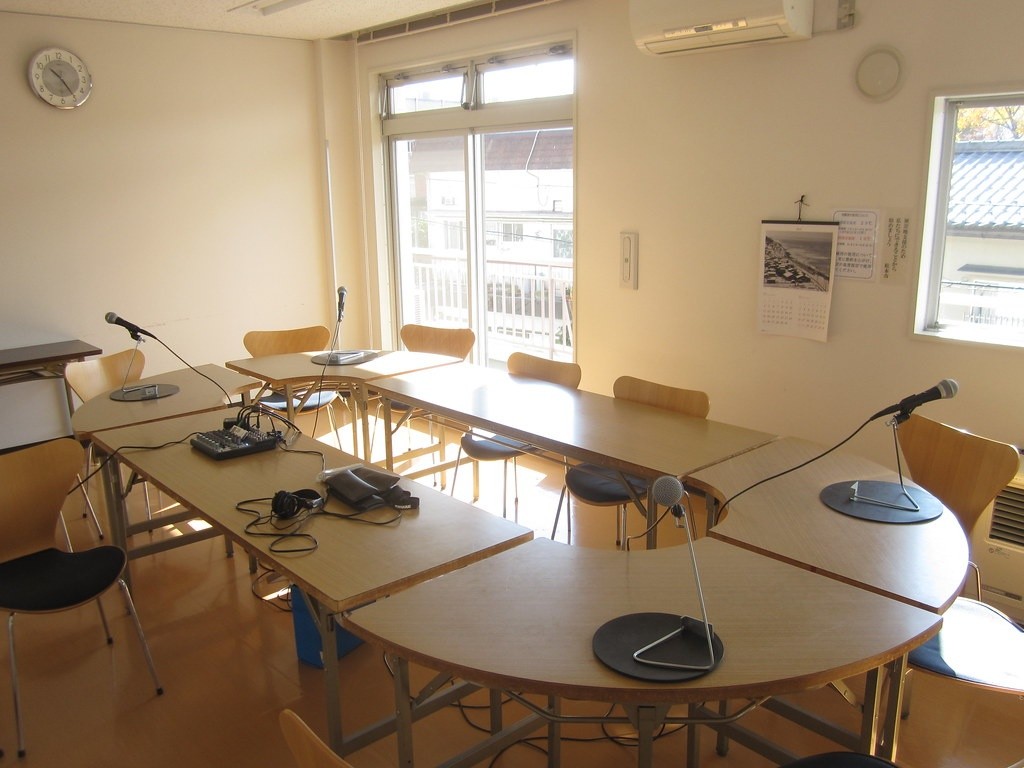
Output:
[27,44,93,109]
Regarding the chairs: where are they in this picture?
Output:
[551,376,710,552]
[880,412,1024,750]
[63,348,163,533]
[448,352,581,532]
[0,437,163,757]
[243,326,341,451]
[280,709,356,768]
[370,323,475,486]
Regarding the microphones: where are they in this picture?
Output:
[105,313,157,340]
[337,286,347,322]
[869,379,959,420]
[653,476,684,508]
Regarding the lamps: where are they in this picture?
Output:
[853,44,906,101]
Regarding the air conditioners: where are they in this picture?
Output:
[629,0,855,57]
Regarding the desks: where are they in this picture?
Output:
[0,339,103,465]
[686,435,970,763]
[227,350,463,486]
[91,406,534,756]
[364,362,780,549]
[345,534,944,767]
[73,363,261,535]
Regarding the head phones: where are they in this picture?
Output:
[272,487,324,519]
